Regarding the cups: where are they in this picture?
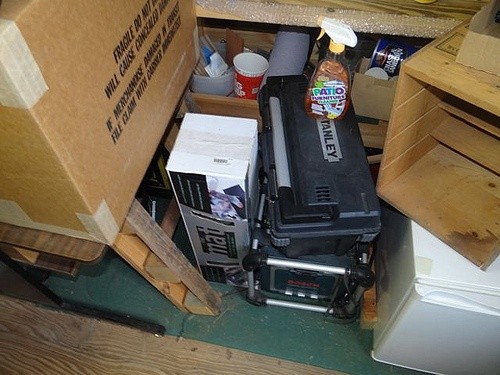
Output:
[233,53,269,100]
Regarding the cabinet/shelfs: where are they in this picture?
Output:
[375,16,500,270]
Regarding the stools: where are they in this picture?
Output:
[0,89,225,317]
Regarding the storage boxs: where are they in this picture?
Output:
[167,111,263,290]
[0,0,202,247]
[353,73,397,122]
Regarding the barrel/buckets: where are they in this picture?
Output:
[188,43,253,96]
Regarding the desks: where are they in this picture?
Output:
[0,293,346,375]
